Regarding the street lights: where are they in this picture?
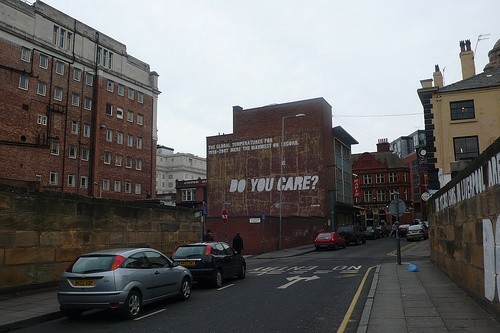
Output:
[279,113,306,249]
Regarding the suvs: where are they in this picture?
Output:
[336,224,367,246]
[169,241,247,289]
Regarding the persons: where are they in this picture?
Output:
[204,230,215,242]
[422,223,428,240]
[231,233,244,254]
[390,222,396,238]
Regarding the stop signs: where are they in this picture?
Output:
[221,209,228,224]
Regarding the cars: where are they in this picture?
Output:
[55,247,193,319]
[313,232,347,251]
[365,226,384,240]
[398,225,410,237]
[405,224,428,242]
